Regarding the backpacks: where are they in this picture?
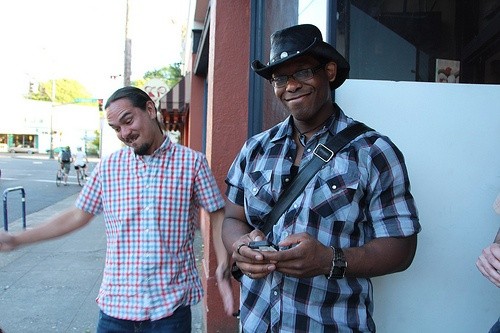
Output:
[62,150,71,163]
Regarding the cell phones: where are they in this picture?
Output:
[248,241,280,252]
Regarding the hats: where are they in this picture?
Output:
[250,24,350,89]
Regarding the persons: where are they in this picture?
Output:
[57,146,72,187]
[220,24,423,333]
[73,146,89,186]
[475,227,499,333]
[0,86,235,333]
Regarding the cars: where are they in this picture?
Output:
[46,146,68,155]
[8,144,38,155]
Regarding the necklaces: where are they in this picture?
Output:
[289,111,334,147]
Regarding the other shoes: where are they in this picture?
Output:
[79,183,82,186]
[84,173,88,177]
[64,182,69,185]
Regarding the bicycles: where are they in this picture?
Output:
[55,160,73,188]
[76,161,90,188]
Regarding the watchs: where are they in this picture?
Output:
[325,246,348,280]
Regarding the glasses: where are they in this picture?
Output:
[270,66,326,88]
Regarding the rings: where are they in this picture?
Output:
[237,244,247,255]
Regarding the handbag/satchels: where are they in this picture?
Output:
[232,121,376,282]
